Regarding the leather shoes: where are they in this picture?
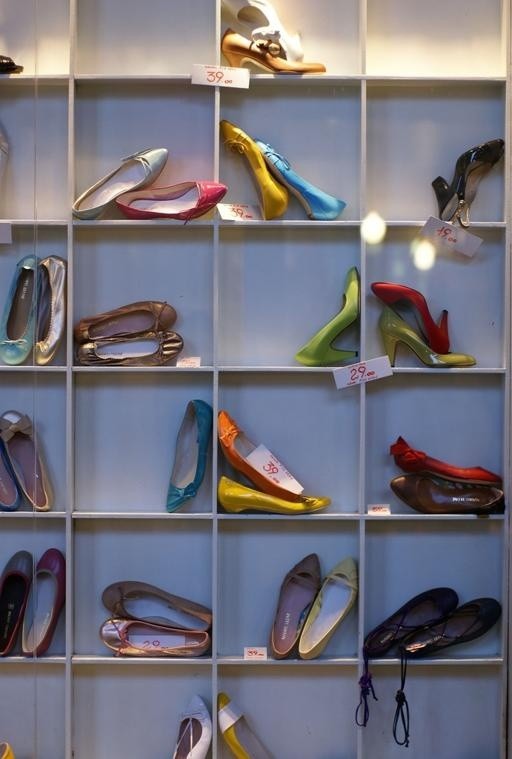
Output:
[366,439,500,657]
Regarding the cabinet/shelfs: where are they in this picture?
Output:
[0,1,512,758]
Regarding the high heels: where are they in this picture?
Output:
[219,0,326,78]
[432,138,504,229]
[299,269,477,369]
